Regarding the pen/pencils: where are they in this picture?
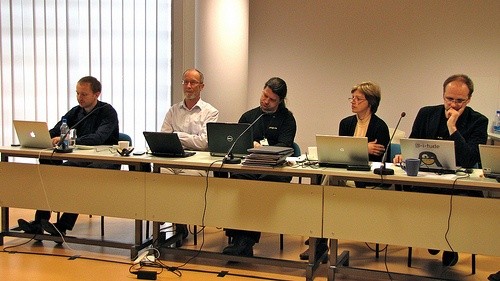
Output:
[480,174,484,177]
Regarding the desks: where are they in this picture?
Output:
[0,145,500,281]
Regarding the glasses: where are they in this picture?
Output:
[444,96,469,105]
[181,80,199,85]
[348,98,365,102]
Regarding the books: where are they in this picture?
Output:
[242,145,294,168]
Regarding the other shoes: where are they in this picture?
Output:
[428,249,440,255]
[41,218,66,245]
[305,237,325,245]
[223,236,255,256]
[442,251,458,266]
[300,246,328,259]
[18,219,44,242]
[487,270,500,281]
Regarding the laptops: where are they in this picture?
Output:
[13,120,59,149]
[478,144,500,178]
[399,138,462,174]
[315,135,369,167]
[205,122,254,158]
[142,131,196,158]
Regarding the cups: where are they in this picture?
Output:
[69,129,76,149]
[308,146,318,161]
[406,158,420,176]
[118,141,129,152]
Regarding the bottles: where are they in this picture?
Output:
[60,119,69,149]
[492,110,500,134]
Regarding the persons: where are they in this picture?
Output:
[300,80,391,259]
[223,77,296,257]
[393,73,488,267]
[159,68,219,248]
[18,75,119,244]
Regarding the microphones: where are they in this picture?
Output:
[374,112,406,175]
[54,103,110,152]
[222,111,273,164]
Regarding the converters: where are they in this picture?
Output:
[136,270,157,280]
